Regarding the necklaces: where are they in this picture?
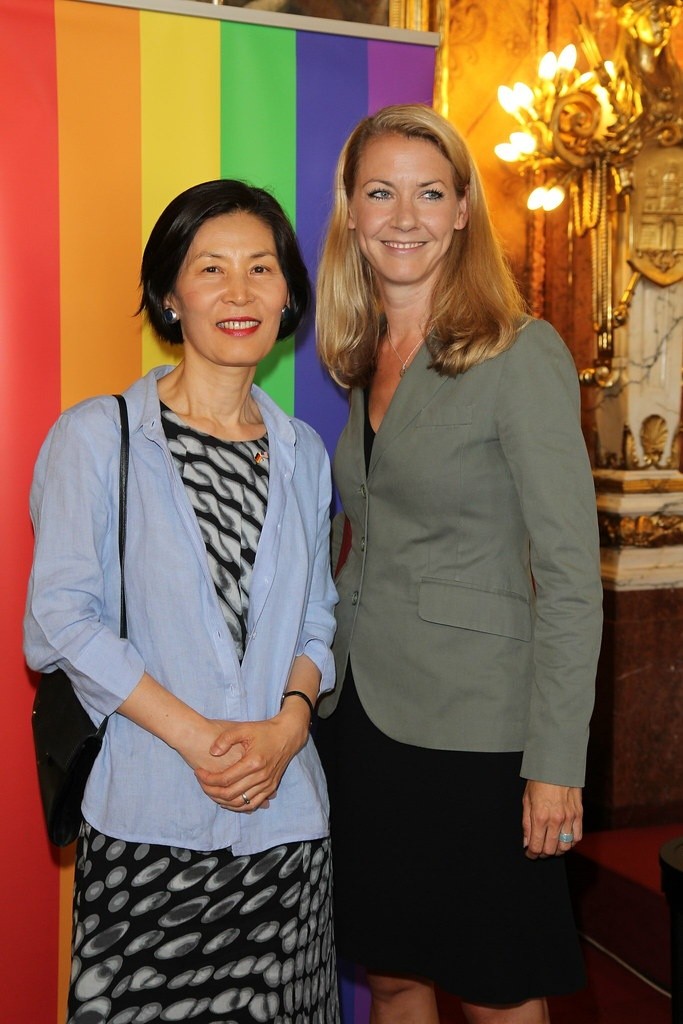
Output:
[386,321,436,377]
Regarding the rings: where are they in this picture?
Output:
[242,793,250,804]
[558,833,573,842]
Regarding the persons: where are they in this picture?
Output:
[23,178,339,1024]
[314,107,602,1024]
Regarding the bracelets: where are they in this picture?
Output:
[281,691,314,727]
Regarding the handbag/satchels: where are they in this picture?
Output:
[32,670,103,846]
